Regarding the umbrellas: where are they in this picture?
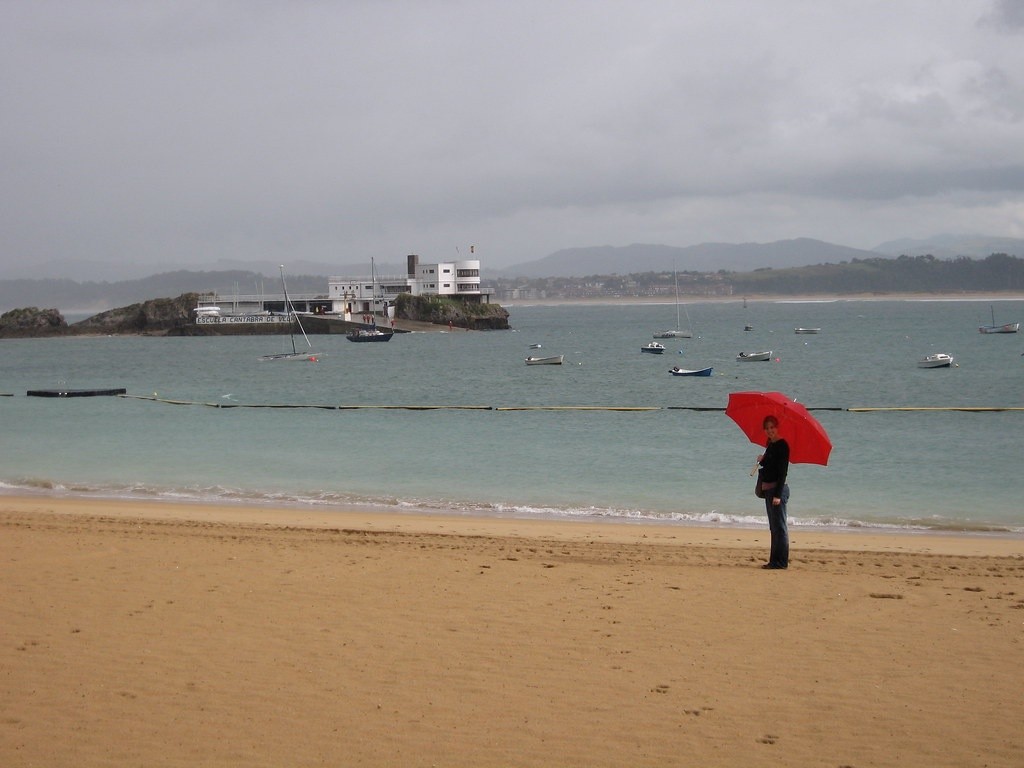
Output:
[724,391,832,477]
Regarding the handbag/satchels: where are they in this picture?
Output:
[755,468,765,498]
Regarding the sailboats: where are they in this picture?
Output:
[653,269,694,338]
[346,256,394,342]
[261,265,322,361]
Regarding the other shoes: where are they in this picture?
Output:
[762,563,779,569]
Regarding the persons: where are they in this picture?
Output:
[449,320,452,329]
[391,319,395,326]
[363,314,374,323]
[755,415,790,569]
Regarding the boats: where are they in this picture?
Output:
[917,354,954,368]
[529,343,541,348]
[794,327,820,334]
[640,342,666,354]
[736,350,772,362]
[525,355,564,366]
[668,366,714,376]
[978,306,1020,334]
